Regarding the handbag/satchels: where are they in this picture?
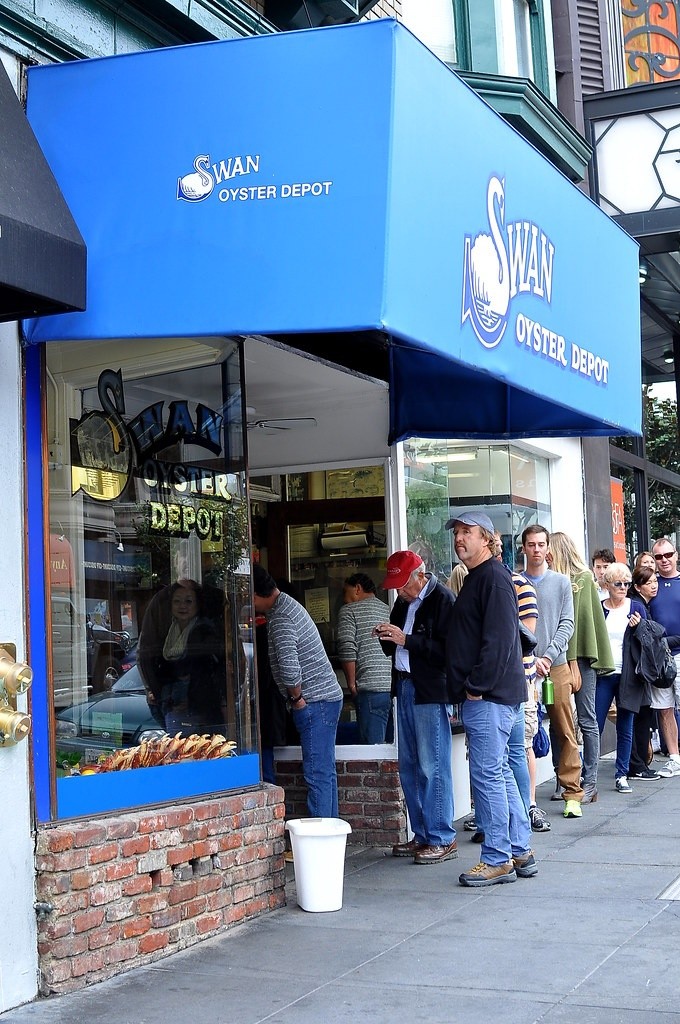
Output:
[651,648,677,688]
[518,619,537,657]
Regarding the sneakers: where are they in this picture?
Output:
[657,759,680,777]
[464,818,476,830]
[528,805,551,831]
[627,769,661,780]
[512,850,538,877]
[616,776,632,793]
[563,800,583,818]
[459,859,517,887]
[414,838,459,864]
[392,838,427,856]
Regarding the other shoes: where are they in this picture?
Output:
[472,833,486,844]
[284,847,295,862]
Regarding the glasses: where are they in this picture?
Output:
[653,551,676,560]
[605,581,631,589]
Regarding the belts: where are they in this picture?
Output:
[397,671,413,680]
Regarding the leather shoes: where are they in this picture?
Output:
[646,732,653,765]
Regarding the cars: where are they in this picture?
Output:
[86,617,143,697]
[53,640,256,770]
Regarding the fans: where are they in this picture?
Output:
[190,405,317,432]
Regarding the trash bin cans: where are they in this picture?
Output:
[285,818,352,912]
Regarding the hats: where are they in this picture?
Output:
[253,563,267,590]
[380,550,422,589]
[445,511,493,535]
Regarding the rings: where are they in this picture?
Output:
[389,631,392,637]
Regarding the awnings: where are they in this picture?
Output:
[0,43,88,323]
[20,17,641,448]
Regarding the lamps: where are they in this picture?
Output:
[320,529,371,548]
[663,350,673,363]
[639,262,650,288]
[414,447,480,464]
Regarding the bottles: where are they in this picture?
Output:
[62,759,81,777]
[542,672,554,705]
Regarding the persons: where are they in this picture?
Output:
[252,563,344,862]
[545,531,613,804]
[334,573,391,744]
[592,540,680,792]
[254,612,292,782]
[145,579,214,717]
[444,562,471,599]
[444,511,551,887]
[371,550,457,864]
[518,524,585,818]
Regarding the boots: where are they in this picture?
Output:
[580,771,597,805]
[551,773,566,799]
